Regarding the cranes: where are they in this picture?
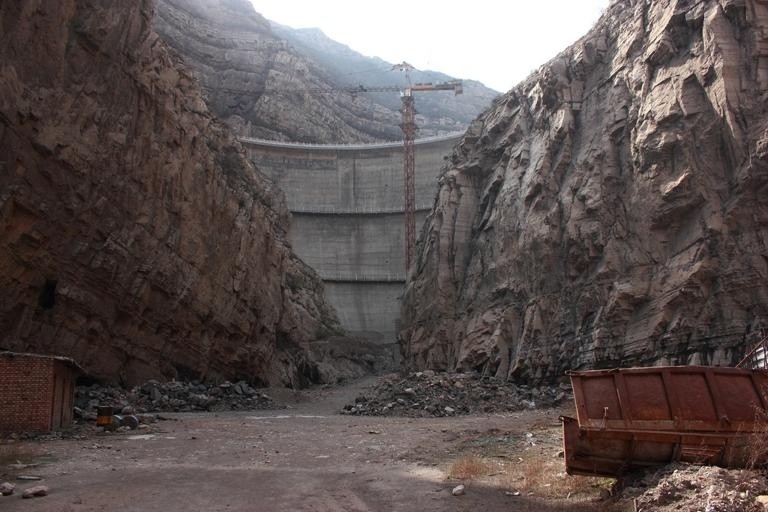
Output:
[201,61,462,280]
